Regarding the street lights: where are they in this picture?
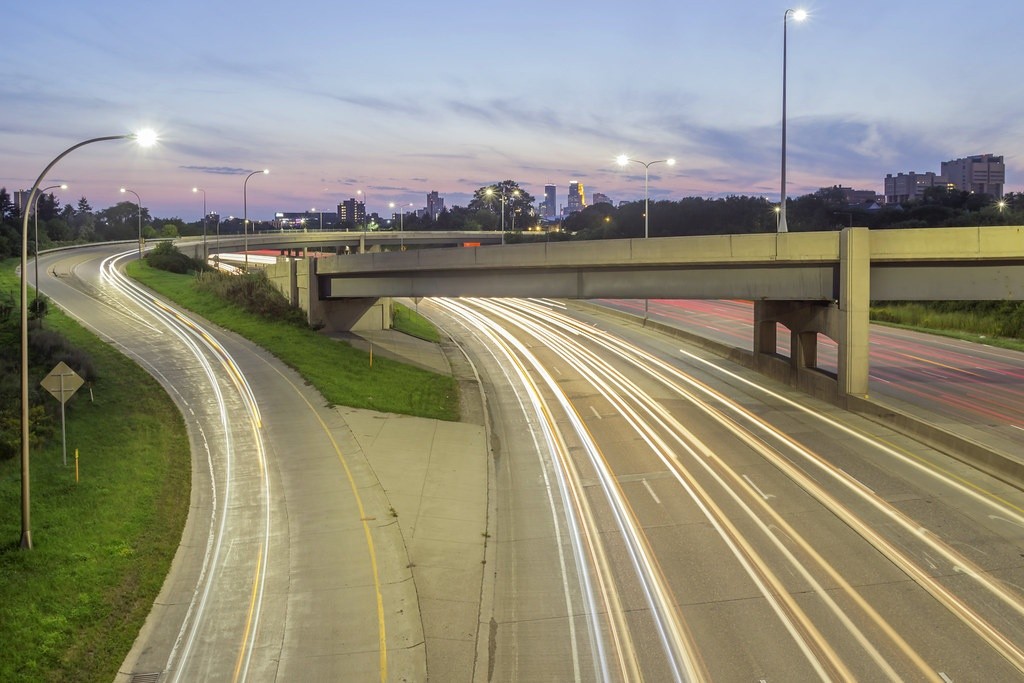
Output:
[17,124,158,554]
[616,156,678,237]
[193,187,210,267]
[34,184,68,310]
[390,201,415,251]
[776,9,808,231]
[120,187,143,260]
[483,188,520,243]
[242,169,271,273]
